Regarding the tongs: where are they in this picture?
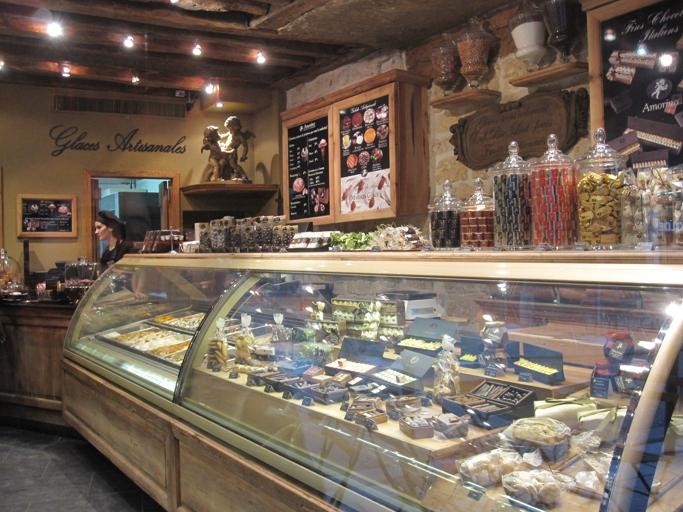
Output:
[537,397,576,410]
[578,406,619,435]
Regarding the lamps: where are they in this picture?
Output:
[60,22,266,94]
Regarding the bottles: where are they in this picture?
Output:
[426,177,494,252]
[494,127,630,253]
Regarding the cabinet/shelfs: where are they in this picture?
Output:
[63,253,683,512]
[579,0,683,173]
[280,69,429,226]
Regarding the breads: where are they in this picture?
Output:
[459,418,572,509]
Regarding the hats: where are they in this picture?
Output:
[94,210,126,229]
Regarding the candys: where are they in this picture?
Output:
[429,166,683,252]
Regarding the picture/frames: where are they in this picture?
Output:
[16,193,77,238]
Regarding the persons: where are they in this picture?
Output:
[210,116,257,183]
[95,210,148,302]
[203,126,235,182]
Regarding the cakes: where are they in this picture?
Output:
[301,146,308,162]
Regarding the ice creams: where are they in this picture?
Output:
[318,139,328,162]
